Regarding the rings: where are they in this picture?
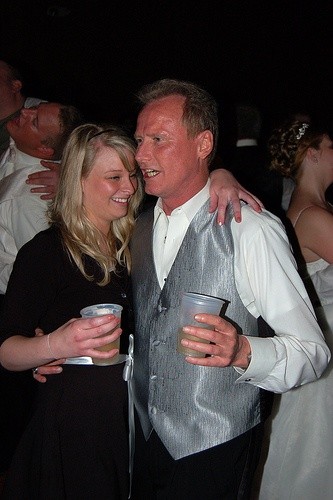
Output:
[32,367,38,373]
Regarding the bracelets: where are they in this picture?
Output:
[46,332,59,360]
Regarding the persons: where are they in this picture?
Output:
[0,123,264,500]
[0,58,86,295]
[31,79,330,500]
[259,119,333,500]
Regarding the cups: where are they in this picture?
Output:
[81,303,122,367]
[176,291,225,359]
